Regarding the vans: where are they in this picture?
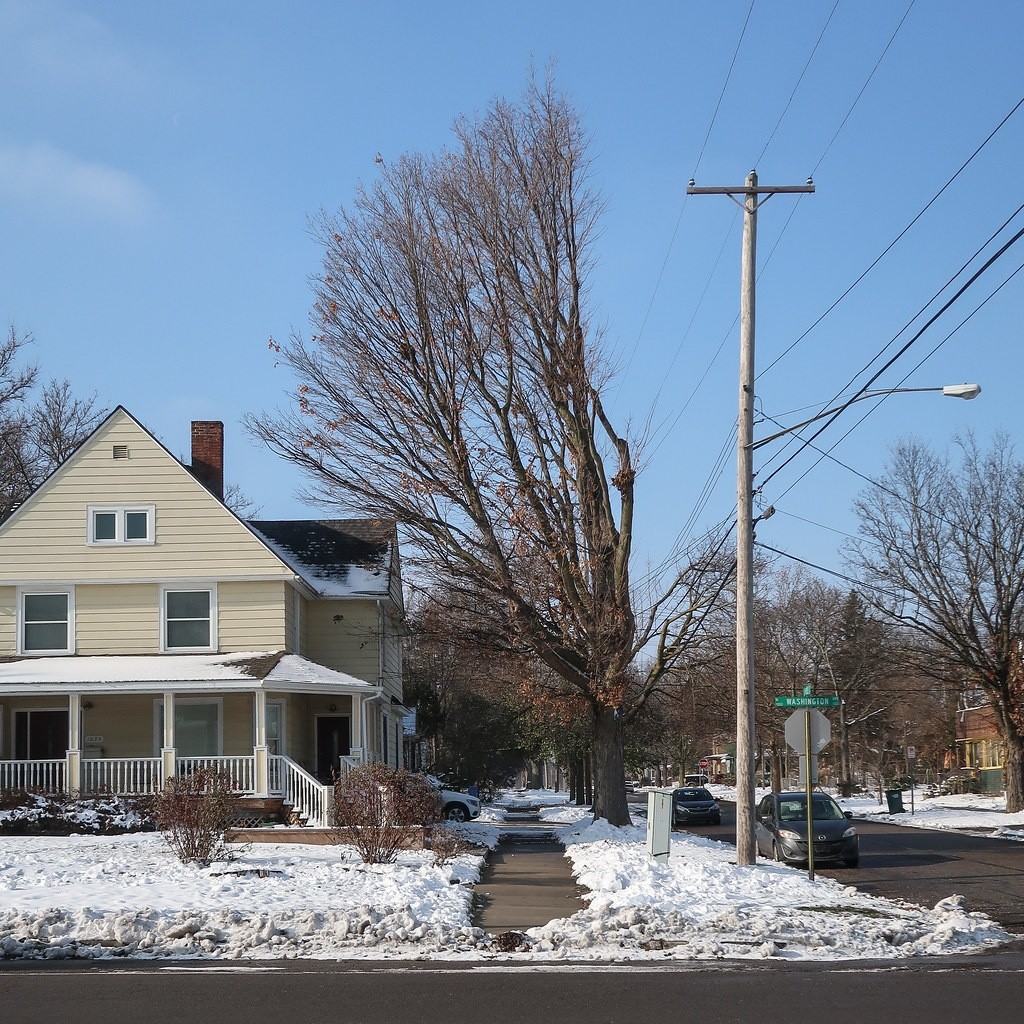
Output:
[683,773,711,788]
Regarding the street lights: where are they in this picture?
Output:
[735,380,983,868]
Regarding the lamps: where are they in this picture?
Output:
[328,704,337,713]
[83,701,92,710]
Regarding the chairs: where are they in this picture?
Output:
[780,806,796,819]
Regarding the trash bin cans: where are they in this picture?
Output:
[886,788,906,814]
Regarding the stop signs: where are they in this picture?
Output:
[698,758,708,768]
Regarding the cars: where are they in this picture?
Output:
[623,780,635,794]
[671,788,723,828]
[427,784,482,824]
[756,792,861,867]
